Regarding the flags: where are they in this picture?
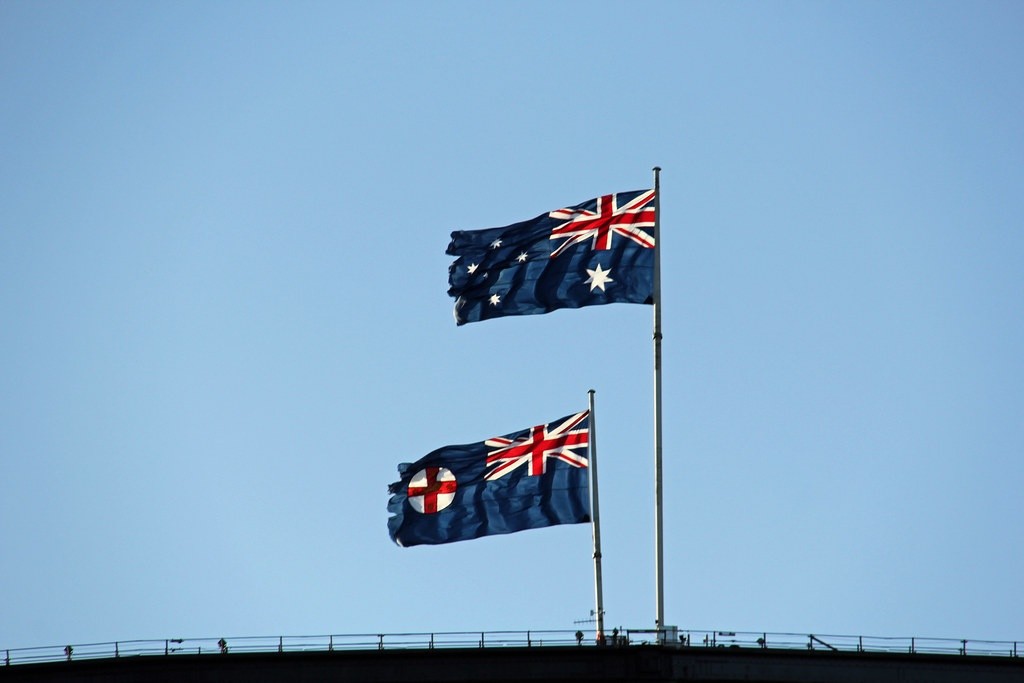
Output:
[445,186,659,326]
[386,408,592,546]
